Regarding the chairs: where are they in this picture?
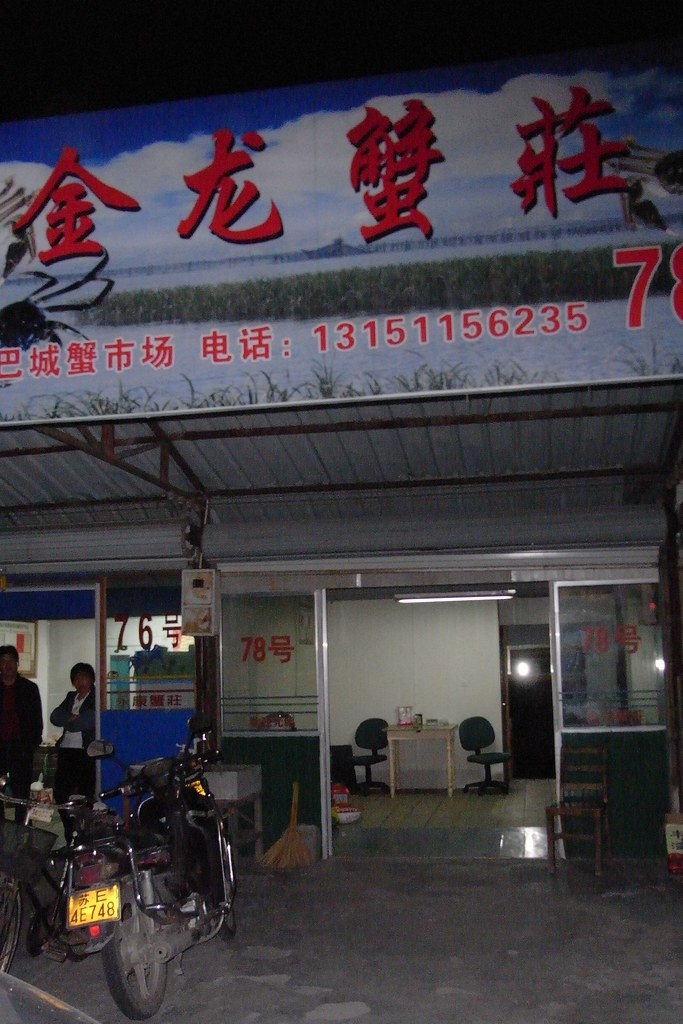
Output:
[544,744,616,878]
[351,718,390,797]
[458,716,513,797]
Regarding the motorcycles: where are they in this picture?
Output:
[63,710,242,1022]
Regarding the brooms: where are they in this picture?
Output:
[260,782,311,871]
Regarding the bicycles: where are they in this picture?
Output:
[1,770,127,976]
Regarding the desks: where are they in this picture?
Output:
[381,723,463,798]
[125,763,263,872]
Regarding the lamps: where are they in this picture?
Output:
[394,588,517,604]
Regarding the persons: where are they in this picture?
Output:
[50,662,98,847]
[0,645,44,835]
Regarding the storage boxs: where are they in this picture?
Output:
[665,813,683,874]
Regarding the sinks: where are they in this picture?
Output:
[129,757,263,800]
